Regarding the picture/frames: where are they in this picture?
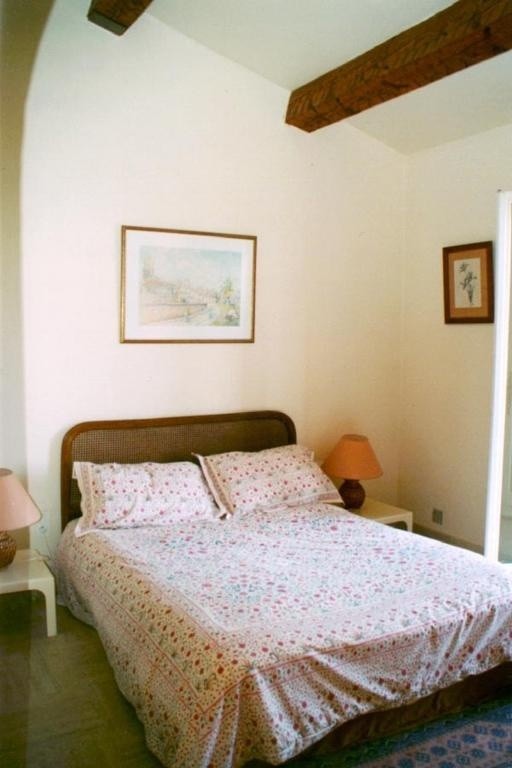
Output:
[442,240,494,324]
[120,224,258,345]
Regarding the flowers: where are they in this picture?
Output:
[458,262,478,302]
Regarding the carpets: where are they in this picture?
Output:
[294,695,512,768]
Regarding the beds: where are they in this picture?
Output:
[59,409,512,768]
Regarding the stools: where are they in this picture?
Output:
[0,548,57,640]
[353,495,413,534]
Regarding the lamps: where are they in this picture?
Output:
[1,467,43,568]
[320,432,383,510]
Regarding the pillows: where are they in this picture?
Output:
[199,445,344,518]
[74,457,219,529]
